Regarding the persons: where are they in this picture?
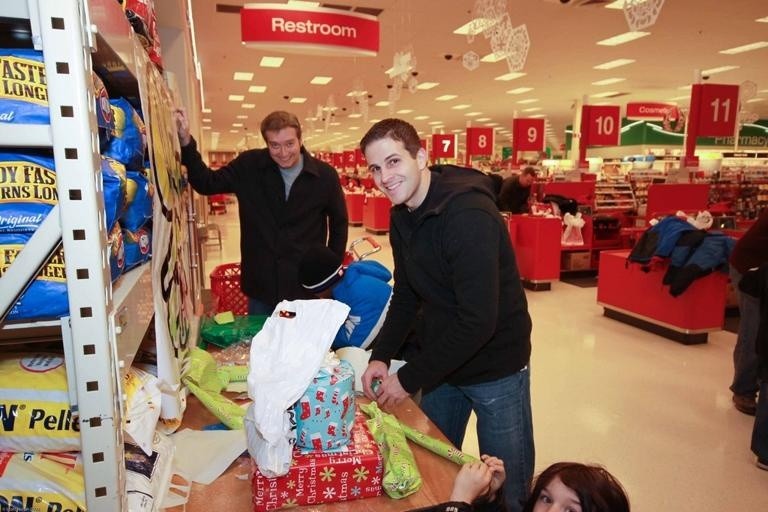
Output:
[444,454,629,512]
[729,206,768,415]
[174,106,348,316]
[738,264,768,470]
[301,248,407,361]
[360,118,535,512]
[499,167,535,212]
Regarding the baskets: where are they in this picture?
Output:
[210,264,247,317]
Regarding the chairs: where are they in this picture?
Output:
[195,195,227,252]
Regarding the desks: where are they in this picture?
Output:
[163,344,466,512]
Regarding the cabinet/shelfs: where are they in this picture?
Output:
[0,0,202,512]
[553,158,767,220]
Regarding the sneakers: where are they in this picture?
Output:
[734,395,757,414]
[756,457,767,469]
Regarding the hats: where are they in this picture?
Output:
[296,245,343,291]
